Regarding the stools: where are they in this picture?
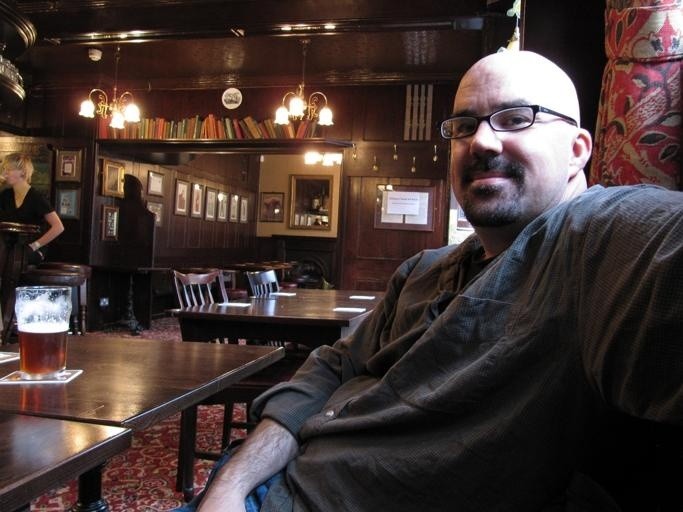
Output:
[220,274,315,301]
[40,261,91,335]
[19,268,84,340]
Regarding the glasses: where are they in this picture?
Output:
[440,104,579,141]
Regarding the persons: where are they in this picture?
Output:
[165,49,683,512]
[0,152,64,344]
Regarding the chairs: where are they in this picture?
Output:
[242,268,309,360]
[171,268,296,462]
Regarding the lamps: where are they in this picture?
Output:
[274,40,335,127]
[371,155,378,171]
[78,44,141,129]
[392,143,398,161]
[410,156,417,173]
[352,143,357,161]
[433,144,439,166]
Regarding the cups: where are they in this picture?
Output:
[13,283,73,382]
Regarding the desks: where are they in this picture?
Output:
[0,411,132,512]
[0,335,287,504]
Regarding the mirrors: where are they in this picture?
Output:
[288,173,334,231]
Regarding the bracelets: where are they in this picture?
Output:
[35,241,41,249]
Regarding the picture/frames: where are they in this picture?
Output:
[100,204,121,241]
[0,142,57,228]
[145,200,166,229]
[53,146,87,186]
[259,191,284,223]
[373,183,437,233]
[146,170,166,199]
[99,158,126,200]
[173,178,249,225]
[53,186,84,225]
[293,210,312,227]
